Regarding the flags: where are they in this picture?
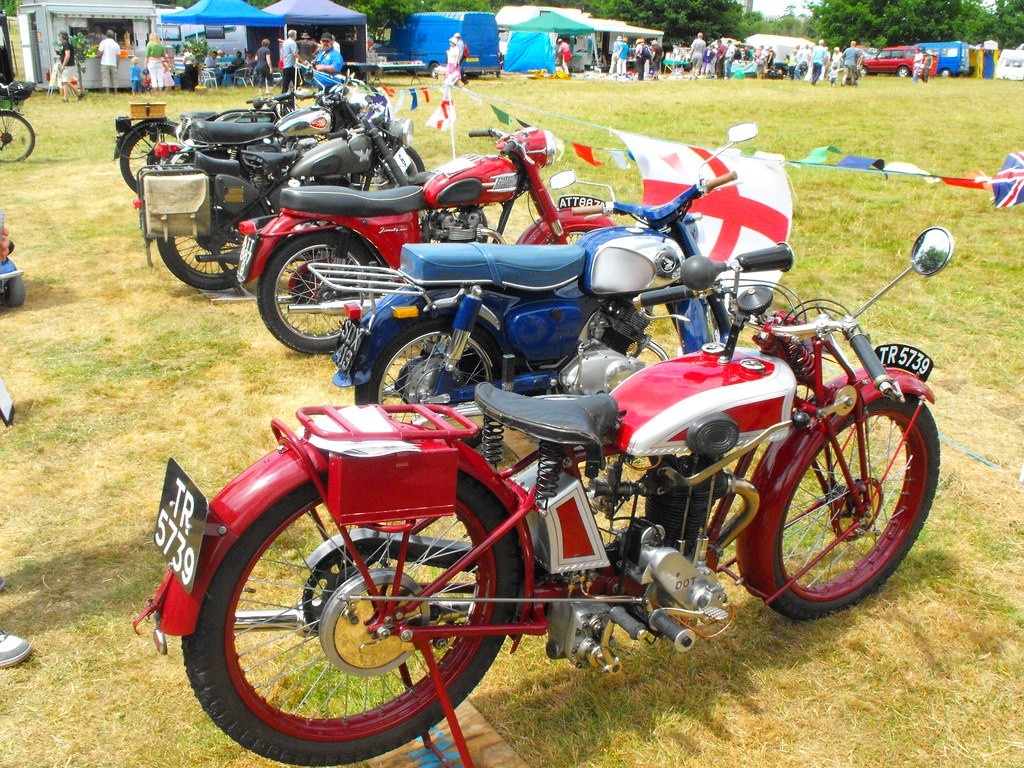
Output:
[490,104,631,170]
[609,126,792,291]
[793,146,1024,208]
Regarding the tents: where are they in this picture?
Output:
[161,0,286,47]
[588,18,664,74]
[746,34,815,63]
[505,11,594,74]
[261,0,369,86]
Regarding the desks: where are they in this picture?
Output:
[663,60,691,74]
[346,61,426,87]
[723,60,760,79]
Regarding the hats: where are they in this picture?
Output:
[57,30,69,39]
[301,32,310,38]
[449,37,457,46]
[558,38,562,43]
[615,36,622,40]
[320,32,332,42]
[454,33,461,38]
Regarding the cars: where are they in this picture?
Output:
[860,46,938,77]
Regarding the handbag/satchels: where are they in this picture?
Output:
[142,173,211,237]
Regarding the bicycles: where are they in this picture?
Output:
[0,73,38,162]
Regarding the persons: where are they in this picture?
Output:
[60,31,85,103]
[281,30,344,94]
[204,49,259,87]
[87,27,121,97]
[445,33,469,88]
[606,36,663,81]
[0,226,34,670]
[910,48,933,83]
[675,33,864,87]
[556,39,573,74]
[256,39,273,96]
[130,33,199,96]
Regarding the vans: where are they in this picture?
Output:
[370,10,501,78]
[906,42,969,78]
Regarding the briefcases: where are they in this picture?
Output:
[129,100,166,118]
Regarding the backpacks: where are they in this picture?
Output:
[643,46,651,58]
[463,43,469,58]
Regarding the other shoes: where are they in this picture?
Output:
[460,79,469,87]
[689,75,698,80]
[77,92,85,102]
[62,99,69,103]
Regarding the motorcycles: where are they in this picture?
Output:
[285,121,761,450]
[237,127,615,355]
[113,59,426,296]
[129,224,955,768]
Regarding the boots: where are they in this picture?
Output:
[651,75,658,79]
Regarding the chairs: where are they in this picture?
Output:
[234,67,253,88]
[199,68,218,90]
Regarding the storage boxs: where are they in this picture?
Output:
[130,102,167,120]
[327,443,460,525]
